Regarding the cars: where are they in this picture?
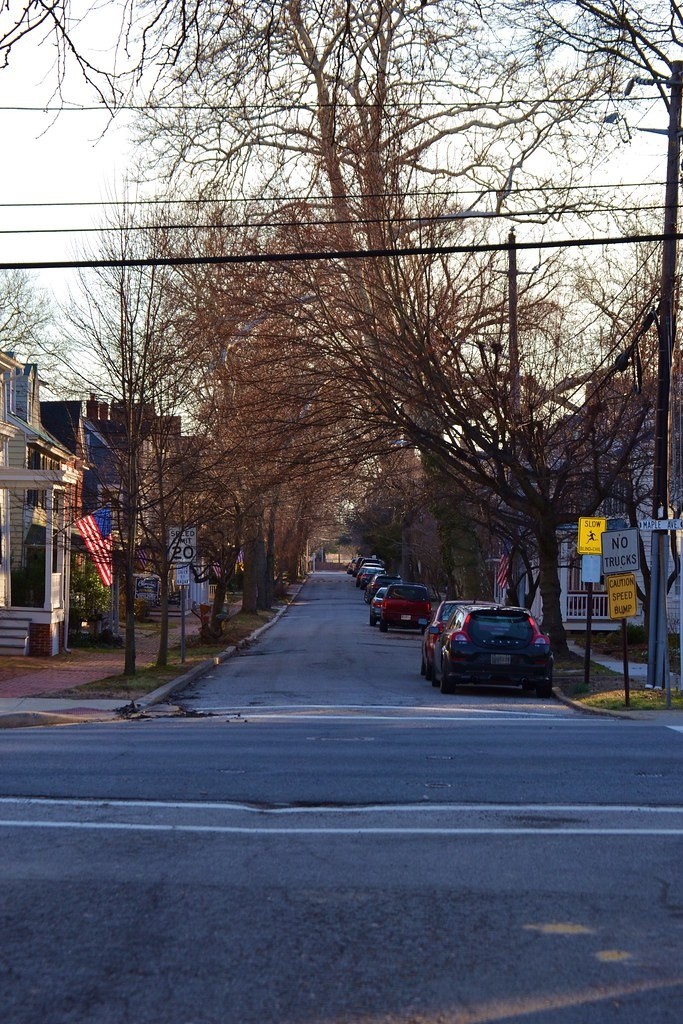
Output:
[369,587,391,626]
[347,556,387,590]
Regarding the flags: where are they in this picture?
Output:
[75,502,121,587]
[497,540,513,588]
[137,549,149,569]
[238,549,244,572]
[212,562,226,578]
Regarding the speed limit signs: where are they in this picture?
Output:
[168,525,196,563]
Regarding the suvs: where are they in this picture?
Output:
[427,605,554,699]
[379,581,436,632]
[417,600,507,681]
[363,572,402,604]
